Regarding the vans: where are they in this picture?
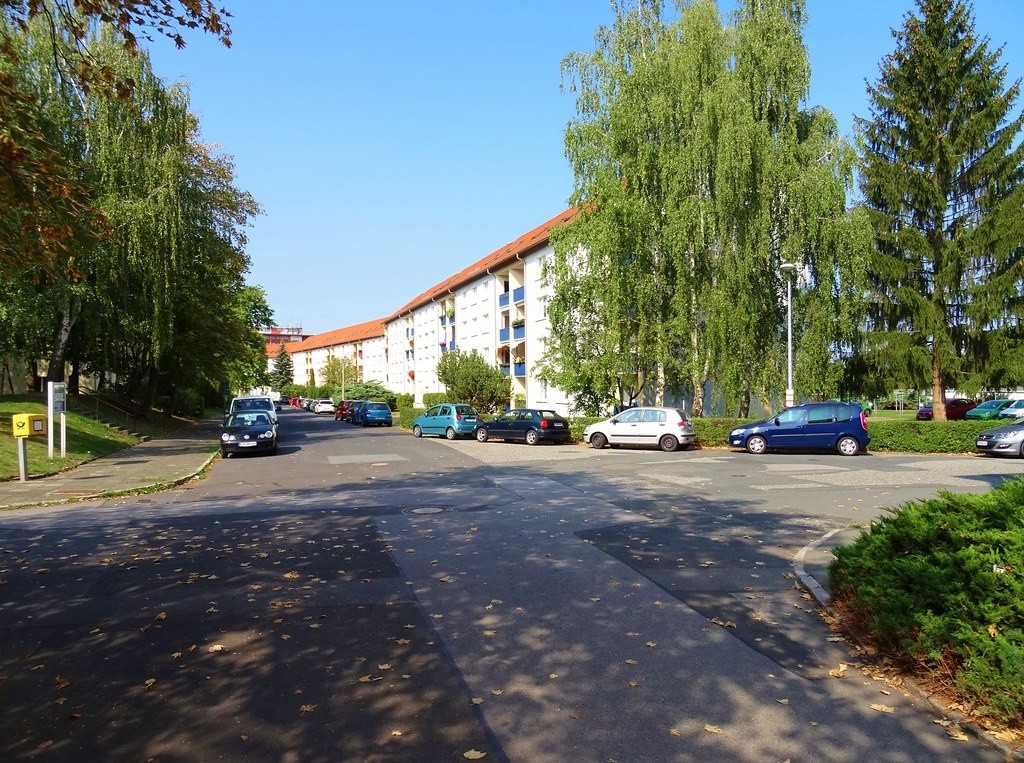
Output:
[268,392,280,403]
[224,396,282,439]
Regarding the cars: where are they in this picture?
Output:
[333,400,352,421]
[219,410,280,459]
[296,399,318,413]
[353,402,393,427]
[411,403,482,440]
[915,397,976,421]
[729,400,871,455]
[315,400,335,415]
[998,399,1024,420]
[279,395,290,404]
[974,419,1024,458]
[288,397,298,407]
[965,400,1022,421]
[825,396,873,417]
[582,406,697,452]
[345,401,364,424]
[472,409,571,444]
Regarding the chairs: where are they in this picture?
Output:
[257,415,266,422]
[236,417,245,425]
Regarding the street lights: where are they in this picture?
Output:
[780,263,796,407]
[340,354,346,401]
